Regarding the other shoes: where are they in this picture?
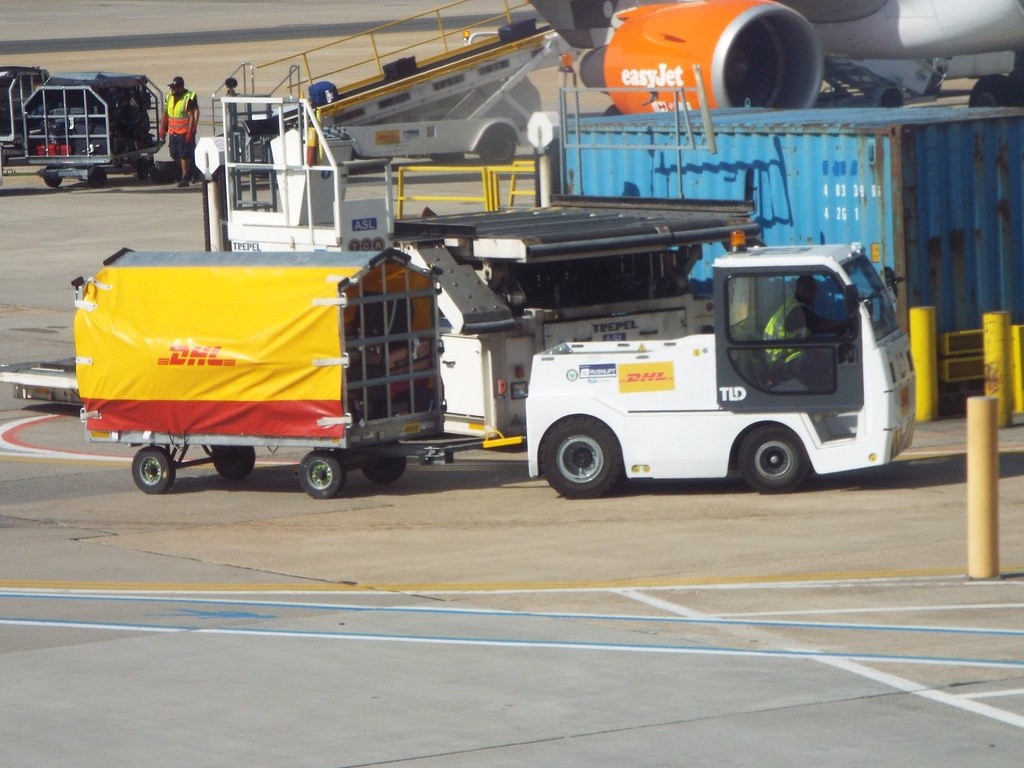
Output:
[174,176,181,182]
[178,181,189,187]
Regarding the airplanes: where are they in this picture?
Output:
[520,0,1024,115]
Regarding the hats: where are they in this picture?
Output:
[167,81,180,87]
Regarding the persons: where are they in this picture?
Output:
[160,78,195,187]
[165,85,200,184]
[762,275,851,388]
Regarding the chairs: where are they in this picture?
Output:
[746,327,767,389]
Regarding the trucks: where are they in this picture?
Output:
[522,227,914,503]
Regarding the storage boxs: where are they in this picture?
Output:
[350,338,433,399]
